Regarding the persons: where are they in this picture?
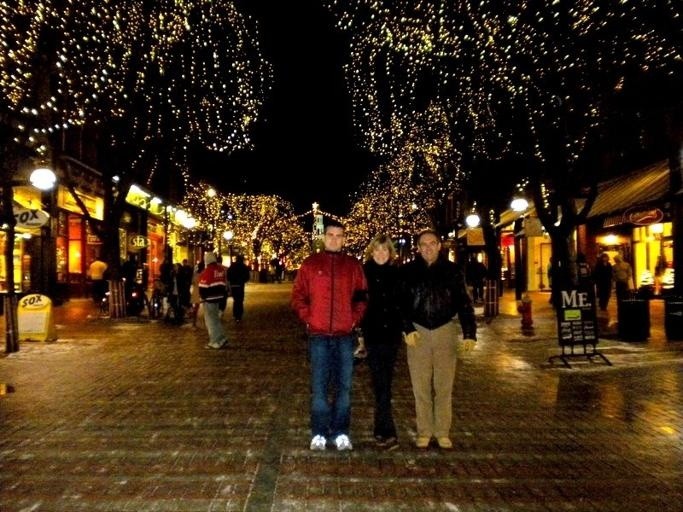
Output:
[400,231,478,448]
[291,221,369,452]
[87,255,107,304]
[463,253,488,303]
[547,256,555,304]
[574,251,634,311]
[119,245,250,350]
[356,233,417,452]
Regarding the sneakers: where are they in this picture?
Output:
[203,336,228,350]
[416,436,430,448]
[330,434,353,451]
[436,436,452,448]
[374,433,400,452]
[309,432,327,451]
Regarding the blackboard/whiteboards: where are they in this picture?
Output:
[554,275,598,343]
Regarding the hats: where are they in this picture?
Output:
[205,252,216,264]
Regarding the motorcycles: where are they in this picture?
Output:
[122,277,144,316]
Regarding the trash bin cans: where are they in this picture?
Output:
[622,299,648,342]
[109,280,127,317]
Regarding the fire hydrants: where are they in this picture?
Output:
[515,291,534,332]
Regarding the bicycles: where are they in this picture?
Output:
[99,280,110,316]
[148,278,160,320]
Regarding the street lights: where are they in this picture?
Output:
[451,211,480,269]
[3,156,56,353]
[173,210,234,264]
[507,196,527,311]
